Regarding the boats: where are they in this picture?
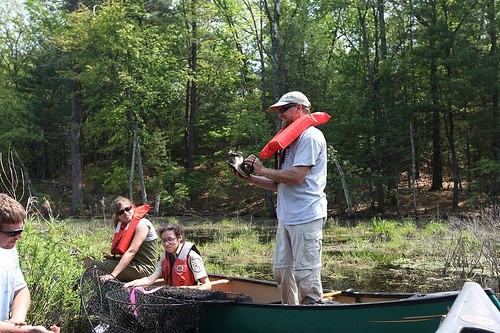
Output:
[114,274,500,333]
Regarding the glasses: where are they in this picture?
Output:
[0,229,23,238]
[278,104,297,113]
[159,237,177,245]
[117,206,132,215]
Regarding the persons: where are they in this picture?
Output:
[0,193,61,333]
[90,195,159,284]
[123,223,212,291]
[228,90,327,301]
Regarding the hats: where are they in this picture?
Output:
[269,90,311,112]
